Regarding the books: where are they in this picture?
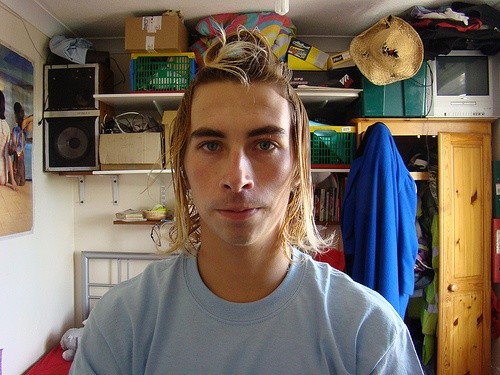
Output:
[115,208,143,220]
[312,177,348,221]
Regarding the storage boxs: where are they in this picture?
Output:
[99,15,358,172]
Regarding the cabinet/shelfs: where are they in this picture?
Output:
[92,88,363,175]
[350,117,495,375]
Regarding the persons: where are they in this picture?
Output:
[68,30,423,375]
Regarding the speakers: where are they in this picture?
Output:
[44,63,112,172]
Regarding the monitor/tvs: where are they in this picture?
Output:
[427,49,500,118]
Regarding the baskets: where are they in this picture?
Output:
[129,52,195,92]
[308,126,358,167]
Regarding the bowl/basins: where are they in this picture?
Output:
[144,210,166,221]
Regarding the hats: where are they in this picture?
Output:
[349,15,424,86]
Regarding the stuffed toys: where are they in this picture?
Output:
[61,327,84,360]
